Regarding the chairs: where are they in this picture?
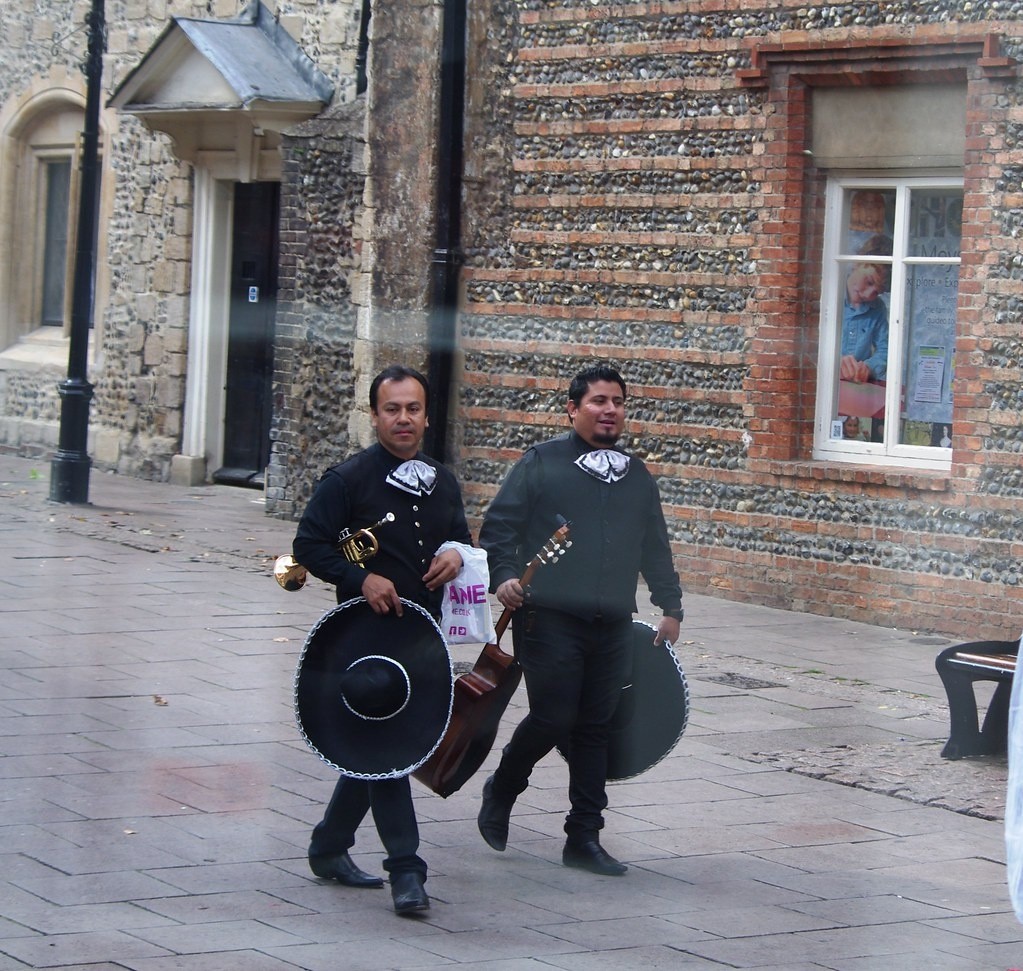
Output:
[936,633,1023,763]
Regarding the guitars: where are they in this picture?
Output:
[410,519,579,801]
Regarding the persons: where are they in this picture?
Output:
[836,233,896,378]
[293,364,486,916]
[843,411,867,440]
[474,363,685,873]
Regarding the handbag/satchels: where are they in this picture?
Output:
[433,540,498,646]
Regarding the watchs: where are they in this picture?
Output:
[659,603,684,621]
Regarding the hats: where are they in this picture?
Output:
[293,597,456,775]
[555,619,688,782]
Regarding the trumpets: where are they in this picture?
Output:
[272,512,395,592]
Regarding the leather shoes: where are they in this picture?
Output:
[563,839,627,873]
[477,775,515,851]
[388,869,430,914]
[308,844,385,889]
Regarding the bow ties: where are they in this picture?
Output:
[385,459,438,497]
[574,449,631,483]
